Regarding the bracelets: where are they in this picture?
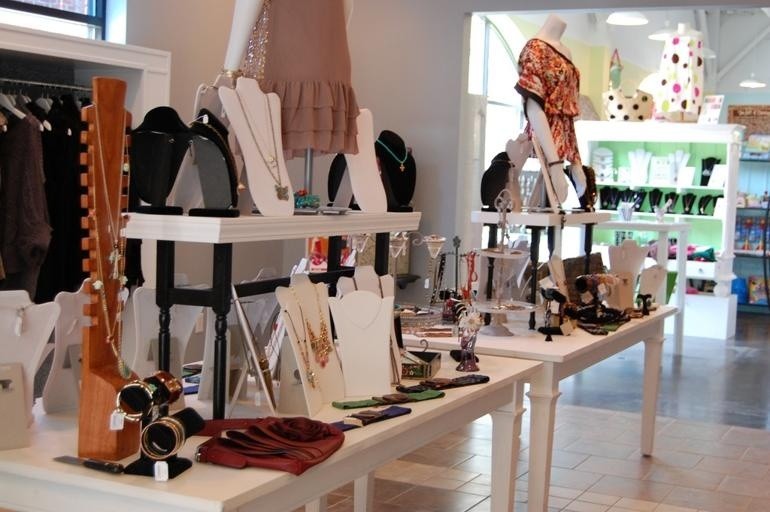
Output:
[220,68,245,81]
[114,368,186,462]
[548,159,565,167]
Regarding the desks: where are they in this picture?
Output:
[1,336,545,512]
[469,204,614,333]
[392,288,681,512]
[116,201,425,421]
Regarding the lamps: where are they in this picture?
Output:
[738,71,768,89]
[605,9,649,26]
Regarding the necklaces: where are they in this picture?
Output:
[351,274,401,383]
[375,136,410,173]
[283,304,317,393]
[92,99,133,381]
[193,119,247,198]
[600,186,710,214]
[232,296,278,412]
[593,152,613,182]
[673,153,685,182]
[233,87,290,201]
[291,282,333,368]
[702,159,719,177]
[10,302,34,338]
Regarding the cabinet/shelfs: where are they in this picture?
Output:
[568,115,742,347]
[733,146,770,318]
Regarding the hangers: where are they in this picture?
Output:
[0,77,89,139]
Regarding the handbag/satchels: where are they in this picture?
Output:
[603,88,652,121]
